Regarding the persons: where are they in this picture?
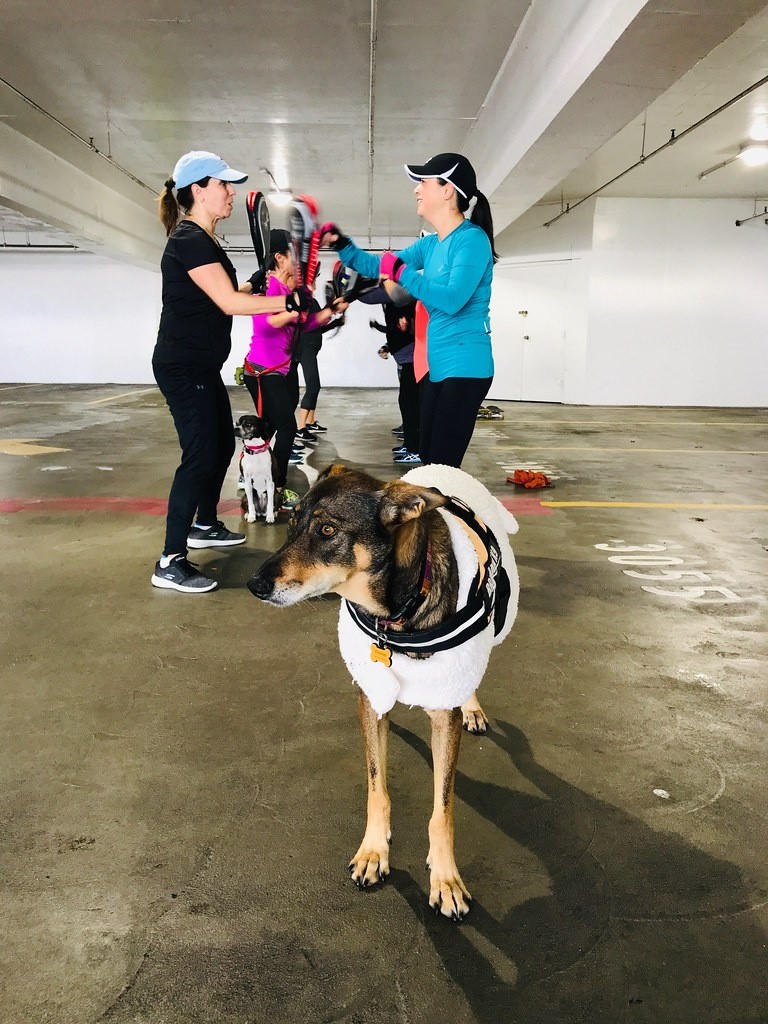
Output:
[151,150,312,594]
[243,229,349,505]
[318,153,499,469]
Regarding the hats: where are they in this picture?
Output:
[404,152,476,201]
[269,229,295,252]
[172,151,248,190]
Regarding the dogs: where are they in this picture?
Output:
[247,463,519,920]
[234,415,280,525]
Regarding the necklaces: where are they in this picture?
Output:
[205,228,219,247]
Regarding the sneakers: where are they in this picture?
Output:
[306,421,328,433]
[288,451,303,463]
[391,445,407,453]
[295,428,317,441]
[238,476,254,489]
[397,433,405,440]
[151,553,217,592]
[293,439,305,450]
[187,520,247,548]
[393,451,421,462]
[391,424,404,433]
[279,487,302,509]
